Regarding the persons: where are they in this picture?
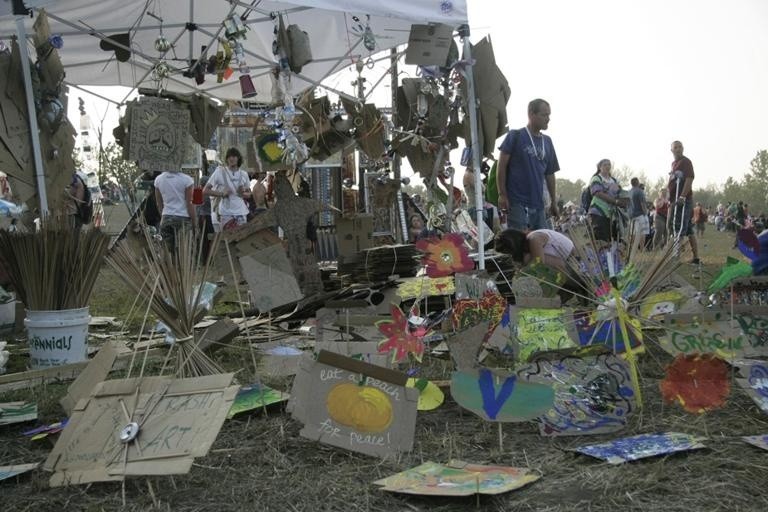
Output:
[628,140,700,264]
[588,158,627,253]
[495,99,561,306]
[751,220,768,277]
[63,158,91,231]
[550,200,768,237]
[496,229,600,306]
[408,166,517,306]
[151,146,315,292]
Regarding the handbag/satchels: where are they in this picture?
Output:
[486,160,511,206]
[699,213,707,222]
[144,195,159,225]
[81,187,92,225]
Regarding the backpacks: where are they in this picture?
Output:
[213,165,241,222]
[582,174,616,212]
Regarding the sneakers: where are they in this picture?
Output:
[690,259,703,266]
